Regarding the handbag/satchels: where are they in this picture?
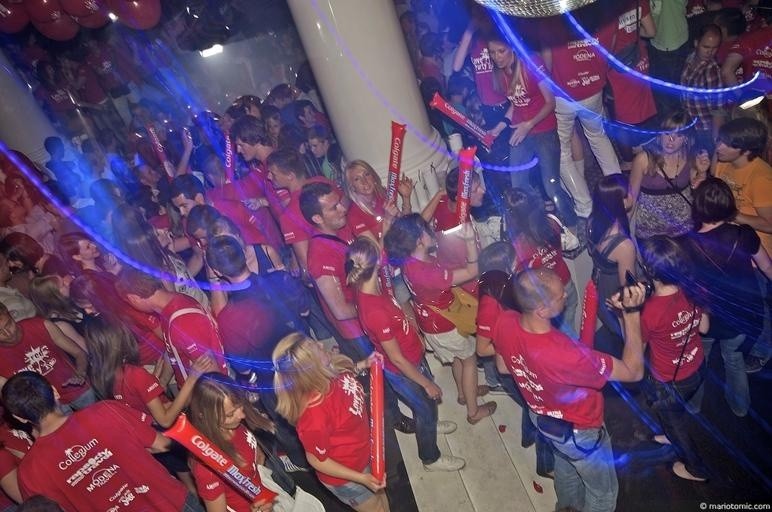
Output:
[440,287,479,335]
[614,43,639,73]
[640,379,685,419]
[561,232,579,252]
[294,486,325,512]
[537,415,574,444]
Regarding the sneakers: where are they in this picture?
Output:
[673,462,706,481]
[437,421,456,434]
[392,415,416,433]
[654,434,673,445]
[744,358,768,373]
[491,384,507,394]
[424,453,464,472]
[724,394,749,417]
[279,454,311,472]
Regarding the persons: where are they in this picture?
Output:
[0,0,772,512]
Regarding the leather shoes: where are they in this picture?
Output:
[466,402,496,424]
[457,386,487,406]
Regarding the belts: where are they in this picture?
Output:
[479,101,509,113]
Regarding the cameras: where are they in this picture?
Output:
[619,269,652,304]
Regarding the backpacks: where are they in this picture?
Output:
[692,227,749,327]
